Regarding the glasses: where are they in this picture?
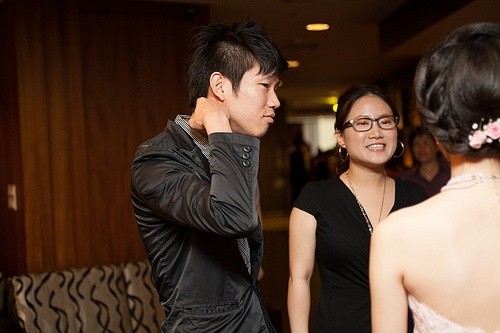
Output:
[339,115,400,132]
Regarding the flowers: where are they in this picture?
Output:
[468,117,500,149]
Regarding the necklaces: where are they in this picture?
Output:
[346,168,386,235]
[441,174,499,191]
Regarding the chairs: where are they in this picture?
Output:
[6,259,165,333]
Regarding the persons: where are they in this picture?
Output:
[368,20,499,333]
[288,131,310,208]
[129,17,288,333]
[405,125,451,198]
[287,84,429,333]
